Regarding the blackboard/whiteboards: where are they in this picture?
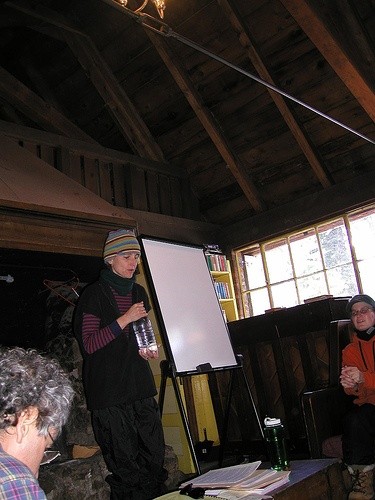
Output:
[137,234,239,377]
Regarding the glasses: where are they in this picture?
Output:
[40,431,62,465]
[179,483,206,499]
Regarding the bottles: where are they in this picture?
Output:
[133,316,158,355]
[263,417,288,472]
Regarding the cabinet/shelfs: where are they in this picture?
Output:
[135,260,351,474]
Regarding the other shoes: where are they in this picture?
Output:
[347,464,375,500]
[66,442,101,460]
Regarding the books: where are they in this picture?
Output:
[207,255,227,271]
[214,282,230,299]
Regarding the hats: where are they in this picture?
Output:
[103,228,141,263]
[343,294,375,311]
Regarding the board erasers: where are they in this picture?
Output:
[196,363,212,373]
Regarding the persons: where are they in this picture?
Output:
[0,346,76,500]
[340,295,375,500]
[74,229,165,500]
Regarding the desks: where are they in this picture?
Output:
[147,458,350,500]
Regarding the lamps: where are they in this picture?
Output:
[118,0,166,19]
